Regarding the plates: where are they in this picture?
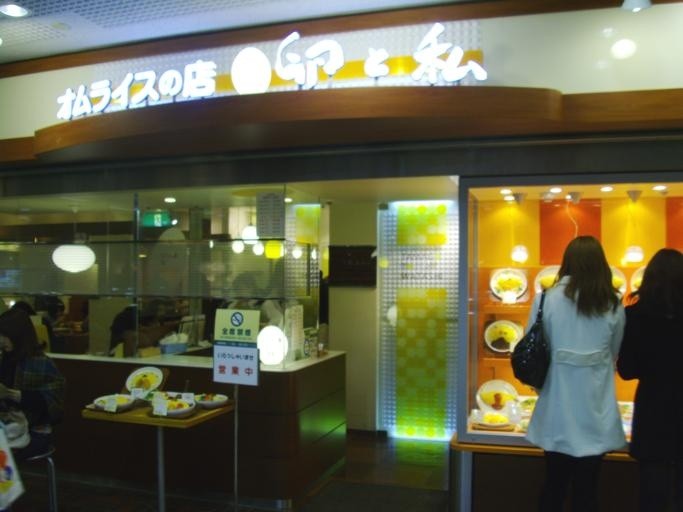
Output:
[480,304,529,368]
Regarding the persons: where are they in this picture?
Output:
[0,309,67,463]
[0,273,285,366]
[523,234,625,512]
[614,247,681,510]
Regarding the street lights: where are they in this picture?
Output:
[0,410,31,449]
[511,289,549,388]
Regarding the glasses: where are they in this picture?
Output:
[52,207,96,272]
[265,240,284,260]
[231,196,259,254]
[511,193,528,262]
[625,190,645,263]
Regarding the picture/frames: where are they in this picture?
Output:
[449,432,638,512]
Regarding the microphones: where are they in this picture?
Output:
[24,446,58,512]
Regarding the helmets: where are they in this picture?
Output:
[482,320,521,352]
[125,365,162,393]
[536,264,647,301]
[470,410,512,427]
[93,393,229,417]
[489,268,527,300]
[256,325,288,365]
[476,379,517,413]
